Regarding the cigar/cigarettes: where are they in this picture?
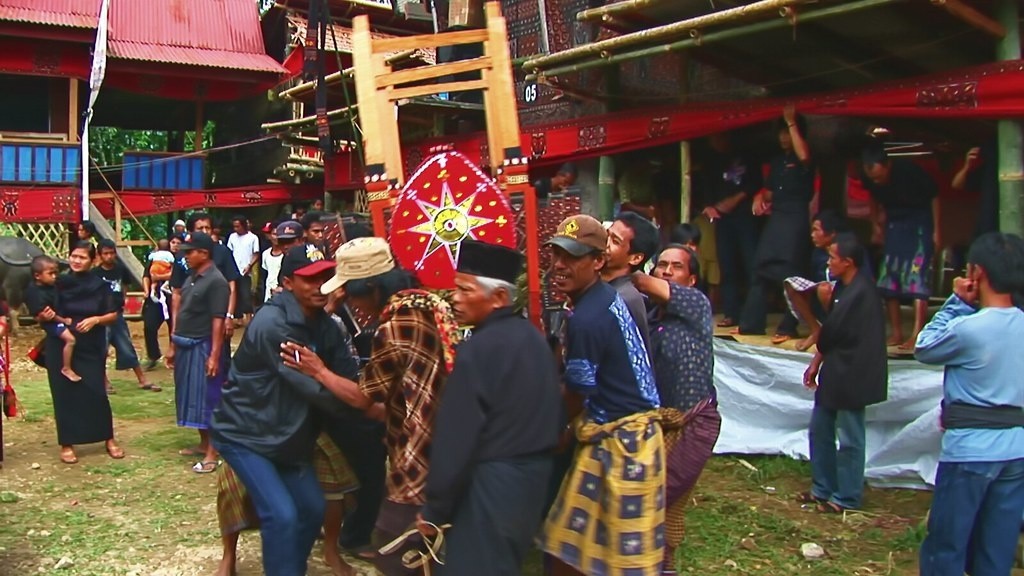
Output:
[711,217,714,223]
[295,350,300,362]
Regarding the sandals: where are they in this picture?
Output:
[796,492,827,503]
[816,501,845,515]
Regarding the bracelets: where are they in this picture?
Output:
[227,314,235,320]
[788,123,797,127]
[95,316,101,324]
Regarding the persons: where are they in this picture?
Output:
[0,99,1024,576]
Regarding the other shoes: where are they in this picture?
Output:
[717,318,737,327]
[146,353,163,371]
[340,544,381,563]
[234,323,243,329]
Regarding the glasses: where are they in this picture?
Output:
[277,238,296,246]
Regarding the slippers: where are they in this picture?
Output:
[106,385,116,394]
[138,383,162,392]
[106,445,126,459]
[178,447,220,456]
[61,450,79,464]
[193,460,224,473]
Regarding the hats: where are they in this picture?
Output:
[277,221,304,238]
[281,243,337,277]
[175,232,214,249]
[320,236,396,296]
[454,239,525,284]
[542,214,607,257]
